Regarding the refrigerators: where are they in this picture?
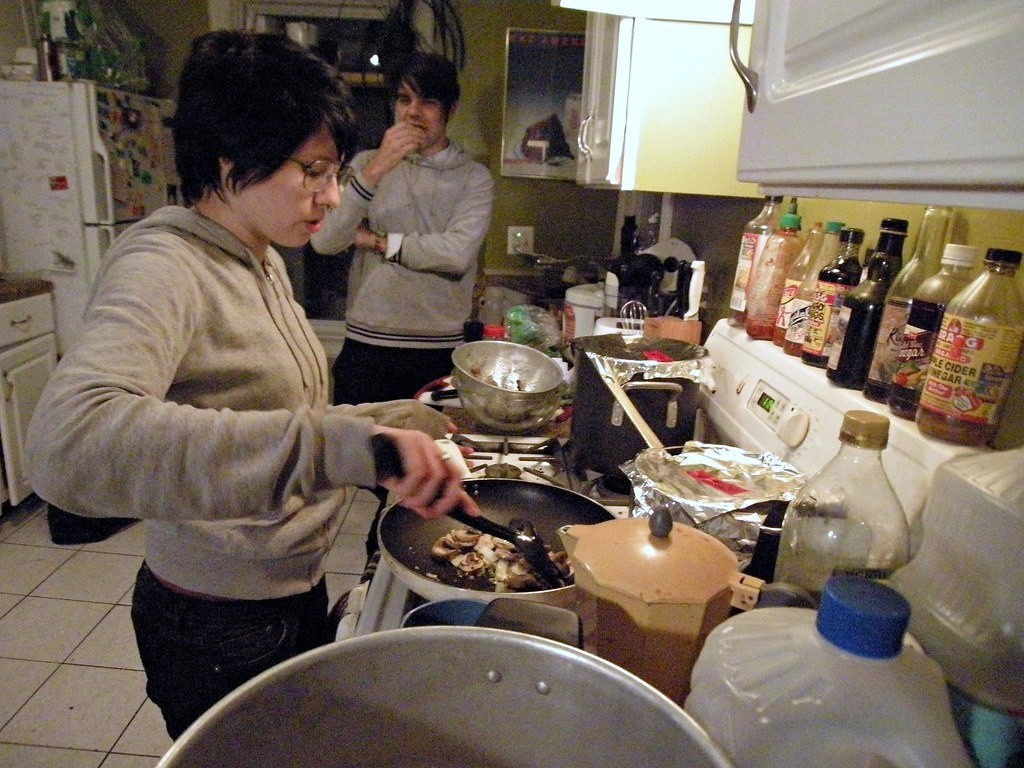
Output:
[0,80,181,355]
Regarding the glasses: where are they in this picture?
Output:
[289,155,355,193]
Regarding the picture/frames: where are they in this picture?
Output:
[499,27,587,178]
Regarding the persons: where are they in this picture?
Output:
[308,51,495,555]
[23,31,480,744]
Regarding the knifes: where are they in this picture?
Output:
[646,258,706,321]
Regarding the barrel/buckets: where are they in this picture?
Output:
[630,444,807,573]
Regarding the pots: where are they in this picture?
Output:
[153,598,734,768]
[572,333,698,475]
[377,439,615,609]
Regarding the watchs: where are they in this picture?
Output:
[373,230,386,253]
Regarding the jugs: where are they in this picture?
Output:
[556,508,818,710]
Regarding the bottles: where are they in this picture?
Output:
[774,411,909,600]
[888,443,1024,768]
[683,576,976,768]
[38,11,60,82]
[728,196,1024,443]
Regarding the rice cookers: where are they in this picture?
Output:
[561,283,606,367]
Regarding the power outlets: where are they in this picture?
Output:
[508,225,534,255]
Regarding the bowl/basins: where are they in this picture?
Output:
[448,341,568,433]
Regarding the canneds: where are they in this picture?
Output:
[563,94,582,139]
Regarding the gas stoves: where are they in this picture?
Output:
[337,317,1024,768]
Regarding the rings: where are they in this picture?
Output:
[440,454,452,462]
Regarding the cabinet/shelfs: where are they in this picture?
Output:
[0,275,59,507]
[550,0,1024,213]
